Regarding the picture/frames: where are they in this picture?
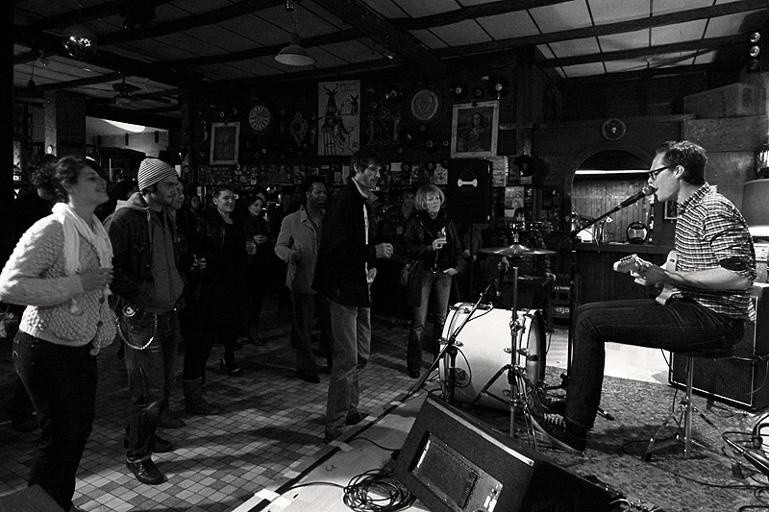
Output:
[209,123,242,164]
[448,100,500,162]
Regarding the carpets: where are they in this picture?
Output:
[525,366,769,511]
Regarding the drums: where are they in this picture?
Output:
[439,301,547,415]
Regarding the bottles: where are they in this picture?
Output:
[526,355,541,385]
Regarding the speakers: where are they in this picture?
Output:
[393,393,622,511]
[669,285,768,412]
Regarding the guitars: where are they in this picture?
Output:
[613,249,677,305]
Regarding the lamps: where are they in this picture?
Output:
[273,0,318,69]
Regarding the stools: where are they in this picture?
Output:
[642,344,746,478]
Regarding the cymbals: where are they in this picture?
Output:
[479,243,556,256]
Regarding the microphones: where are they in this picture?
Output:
[616,183,656,209]
[726,437,769,475]
[495,258,509,297]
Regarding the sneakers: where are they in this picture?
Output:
[524,405,589,453]
[347,412,370,426]
[538,388,570,412]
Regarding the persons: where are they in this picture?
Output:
[114,176,334,427]
[101,157,184,484]
[373,185,463,378]
[2,157,114,508]
[461,112,491,152]
[522,139,756,455]
[312,152,394,443]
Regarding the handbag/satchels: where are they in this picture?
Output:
[398,258,425,307]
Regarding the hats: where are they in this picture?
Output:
[136,156,178,190]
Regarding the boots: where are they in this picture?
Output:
[180,376,222,416]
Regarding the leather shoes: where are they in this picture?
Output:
[218,358,247,377]
[124,455,166,485]
[409,370,421,379]
[152,436,175,454]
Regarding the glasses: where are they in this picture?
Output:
[648,162,684,181]
